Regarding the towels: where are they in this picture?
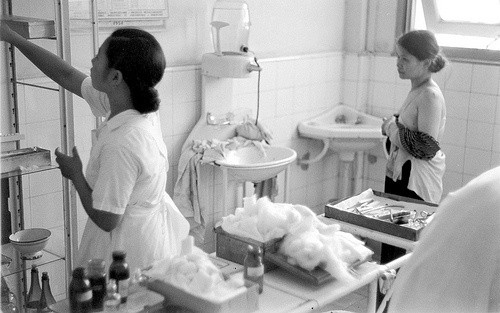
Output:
[173,138,225,244]
[226,118,273,150]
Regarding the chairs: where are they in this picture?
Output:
[376,162,500,313]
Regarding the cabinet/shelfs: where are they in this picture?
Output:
[0,0,77,313]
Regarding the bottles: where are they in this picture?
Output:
[86,258,107,312]
[109,248,129,305]
[126,264,149,311]
[243,244,264,295]
[103,277,121,313]
[69,267,93,313]
[0,274,19,313]
[37,271,56,313]
[25,267,42,313]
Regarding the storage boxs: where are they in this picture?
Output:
[134,265,260,313]
[213,226,369,286]
[325,190,439,242]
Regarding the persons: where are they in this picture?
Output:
[0,23,191,285]
[377,29,445,309]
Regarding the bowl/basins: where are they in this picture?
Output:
[8,228,52,260]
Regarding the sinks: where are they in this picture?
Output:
[297,104,389,162]
[209,144,297,184]
